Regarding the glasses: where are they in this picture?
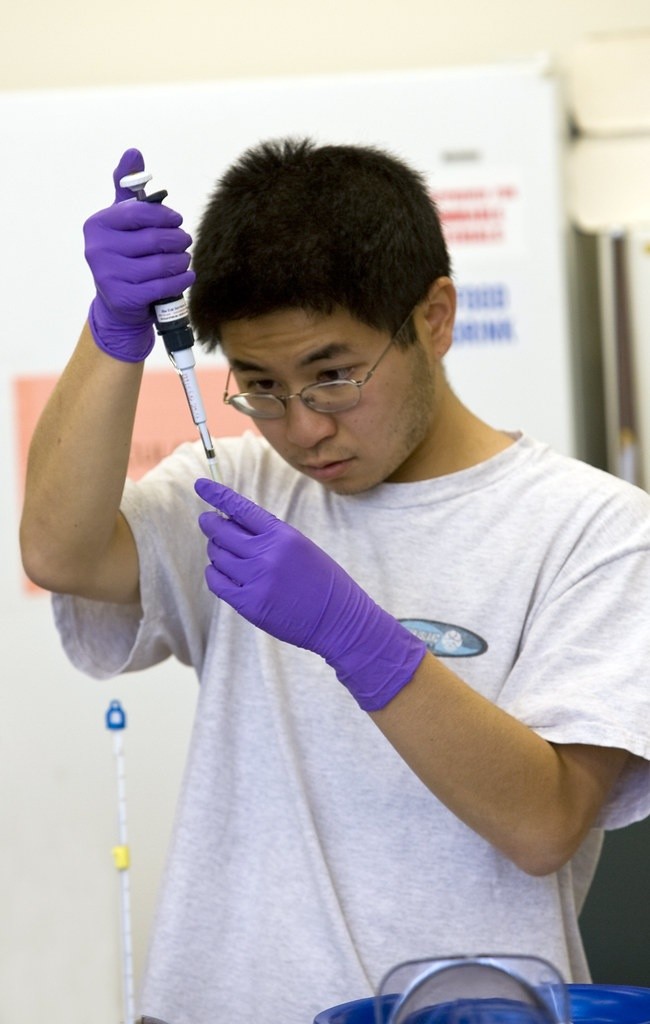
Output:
[222,306,417,419]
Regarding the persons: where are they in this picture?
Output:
[20,143,650,1024]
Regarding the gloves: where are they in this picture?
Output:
[83,148,196,362]
[194,477,426,711]
